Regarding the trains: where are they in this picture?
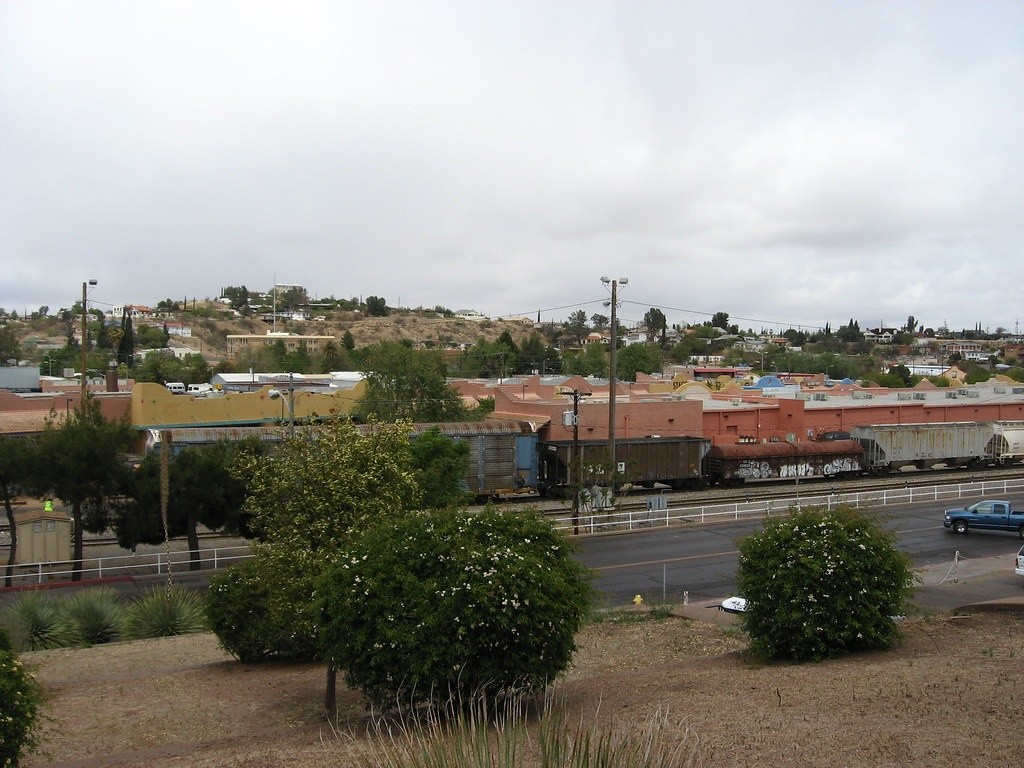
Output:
[112,418,1024,521]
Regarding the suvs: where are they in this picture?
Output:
[1013,544,1024,577]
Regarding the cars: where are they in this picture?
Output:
[314,316,327,322]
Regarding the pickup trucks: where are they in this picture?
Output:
[944,500,1024,541]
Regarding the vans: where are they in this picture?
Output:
[187,383,225,397]
[166,382,185,394]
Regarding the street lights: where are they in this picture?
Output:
[269,373,336,441]
[80,279,98,395]
[825,364,834,375]
[761,351,769,372]
[600,276,629,488]
[543,357,550,375]
[125,354,133,392]
[42,353,51,377]
